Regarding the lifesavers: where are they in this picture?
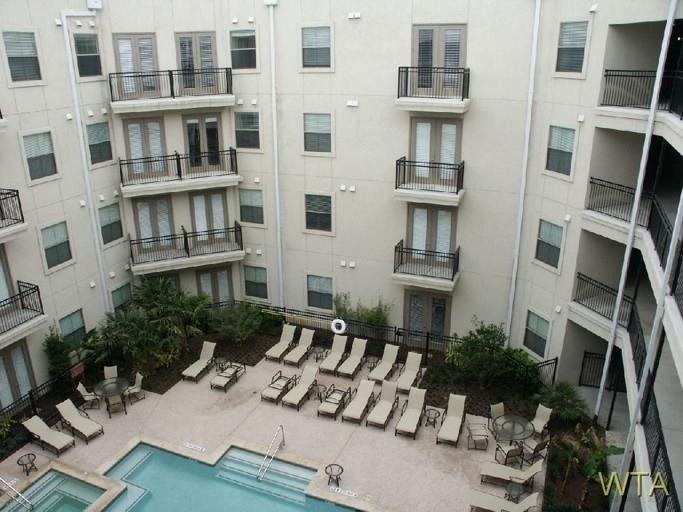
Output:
[331,319,346,334]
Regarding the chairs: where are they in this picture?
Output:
[179,323,554,512]
[20,363,144,459]
